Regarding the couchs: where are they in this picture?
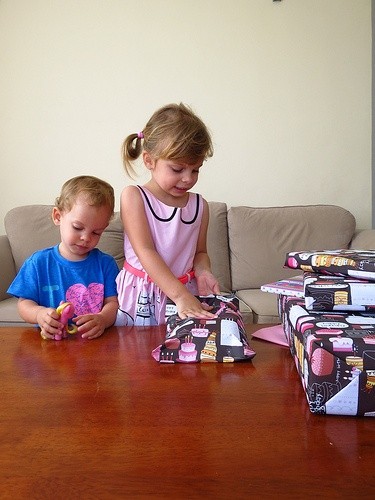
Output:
[0,204,375,328]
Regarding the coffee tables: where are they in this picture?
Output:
[0,326,375,500]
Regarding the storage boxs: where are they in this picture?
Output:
[260,249,375,418]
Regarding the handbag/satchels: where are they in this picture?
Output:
[151,294,256,363]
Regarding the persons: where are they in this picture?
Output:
[6,175,120,340]
[114,102,220,326]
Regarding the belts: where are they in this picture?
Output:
[123,260,195,285]
